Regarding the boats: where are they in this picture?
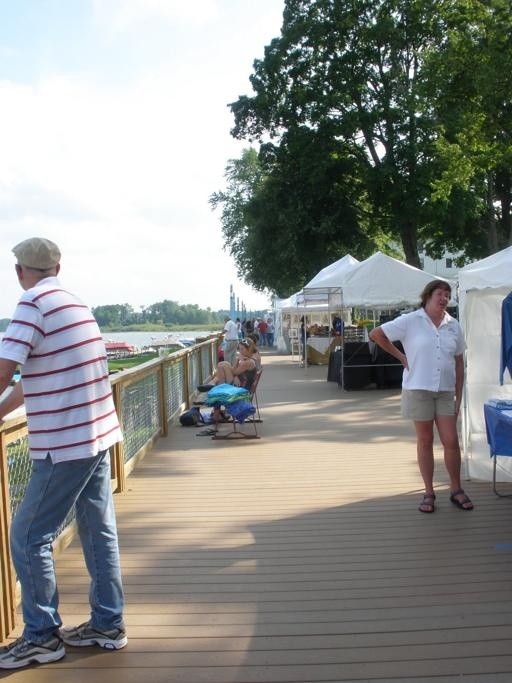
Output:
[104,337,196,356]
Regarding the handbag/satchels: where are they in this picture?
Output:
[180,407,204,426]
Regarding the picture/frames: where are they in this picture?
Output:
[287,327,298,339]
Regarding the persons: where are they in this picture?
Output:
[217,336,225,362]
[299,316,314,366]
[330,312,345,336]
[365,279,474,515]
[218,314,238,366]
[0,236,128,670]
[195,339,256,392]
[236,316,276,370]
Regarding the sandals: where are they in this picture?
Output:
[418,493,436,513]
[449,488,473,510]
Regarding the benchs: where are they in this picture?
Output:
[211,364,263,441]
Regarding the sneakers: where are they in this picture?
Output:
[0,635,66,669]
[62,621,128,650]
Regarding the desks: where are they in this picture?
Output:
[484,405,512,497]
[307,336,341,365]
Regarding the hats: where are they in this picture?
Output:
[11,237,61,269]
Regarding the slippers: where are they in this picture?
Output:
[196,428,218,436]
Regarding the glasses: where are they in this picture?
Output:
[242,339,249,346]
